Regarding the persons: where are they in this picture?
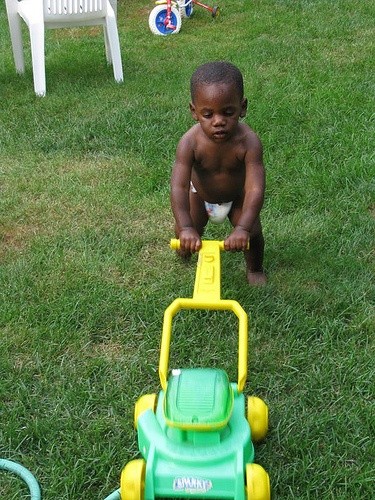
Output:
[167,61,268,288]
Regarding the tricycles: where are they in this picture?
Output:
[148,0,220,36]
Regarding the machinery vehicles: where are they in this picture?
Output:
[120,239,271,500]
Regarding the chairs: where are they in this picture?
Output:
[4,0,124,98]
